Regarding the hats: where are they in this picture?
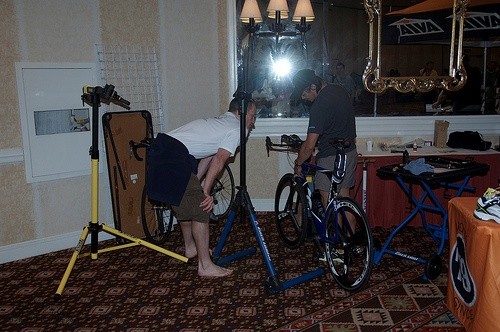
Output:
[290,69,315,99]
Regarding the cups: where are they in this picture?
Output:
[367,141,374,151]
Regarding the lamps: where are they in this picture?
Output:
[214,0,324,294]
[239,10,313,118]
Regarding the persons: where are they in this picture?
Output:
[146,98,257,277]
[432,55,500,115]
[290,70,358,263]
[419,61,438,76]
[326,63,357,102]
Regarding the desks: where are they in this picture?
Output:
[350,147,500,228]
[103,111,165,245]
[373,156,490,282]
[445,196,500,332]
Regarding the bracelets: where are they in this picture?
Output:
[295,160,301,167]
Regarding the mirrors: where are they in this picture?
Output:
[226,0,500,136]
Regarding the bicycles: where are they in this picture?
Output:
[129,132,237,246]
[265,134,374,293]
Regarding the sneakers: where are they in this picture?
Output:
[319,250,348,263]
[472,195,500,224]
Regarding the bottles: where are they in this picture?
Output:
[413,144,417,152]
